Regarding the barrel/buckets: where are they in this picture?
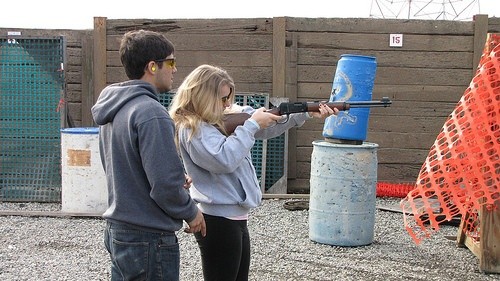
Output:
[306,138,378,249]
[59,127,110,215]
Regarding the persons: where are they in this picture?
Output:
[169,64,339,281]
[91,31,206,281]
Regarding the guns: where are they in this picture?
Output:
[223,96,392,136]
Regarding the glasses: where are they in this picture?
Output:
[221,96,231,102]
[144,59,177,70]
